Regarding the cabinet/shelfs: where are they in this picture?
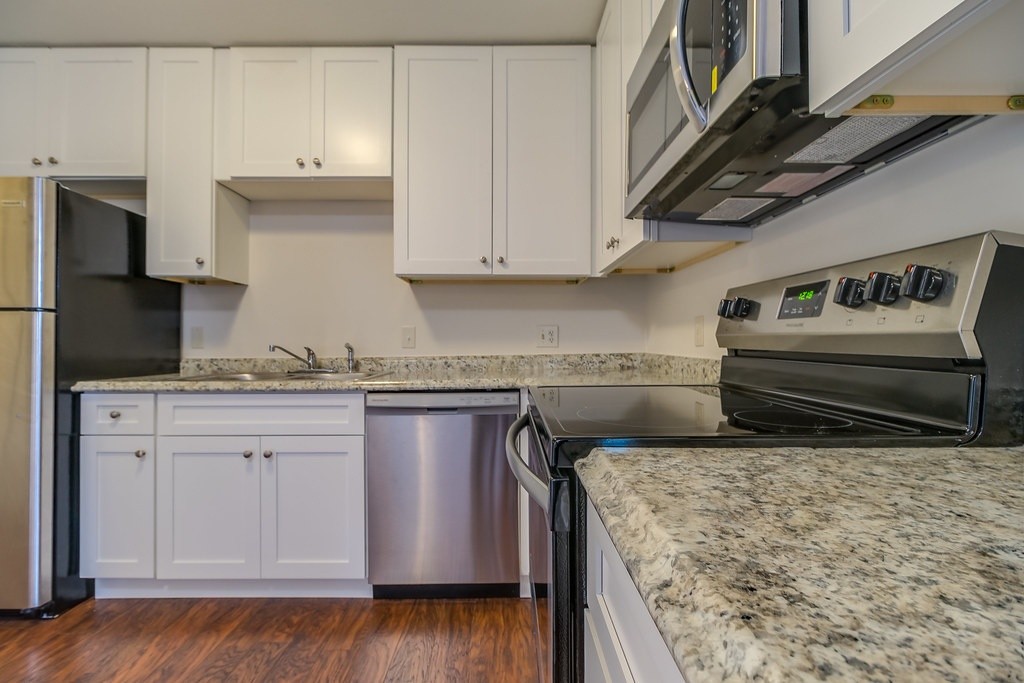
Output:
[807,0,1024,118]
[223,47,394,203]
[79,390,157,599]
[580,490,689,683]
[157,391,374,598]
[593,0,752,278]
[394,44,594,284]
[146,47,250,287]
[0,45,149,182]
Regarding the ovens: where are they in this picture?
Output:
[506,412,574,683]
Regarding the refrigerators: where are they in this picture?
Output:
[0,176,181,612]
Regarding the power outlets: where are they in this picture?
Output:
[536,324,558,347]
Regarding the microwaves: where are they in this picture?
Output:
[625,0,805,224]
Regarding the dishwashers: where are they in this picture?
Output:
[367,391,550,597]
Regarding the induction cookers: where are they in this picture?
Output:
[528,232,1024,466]
